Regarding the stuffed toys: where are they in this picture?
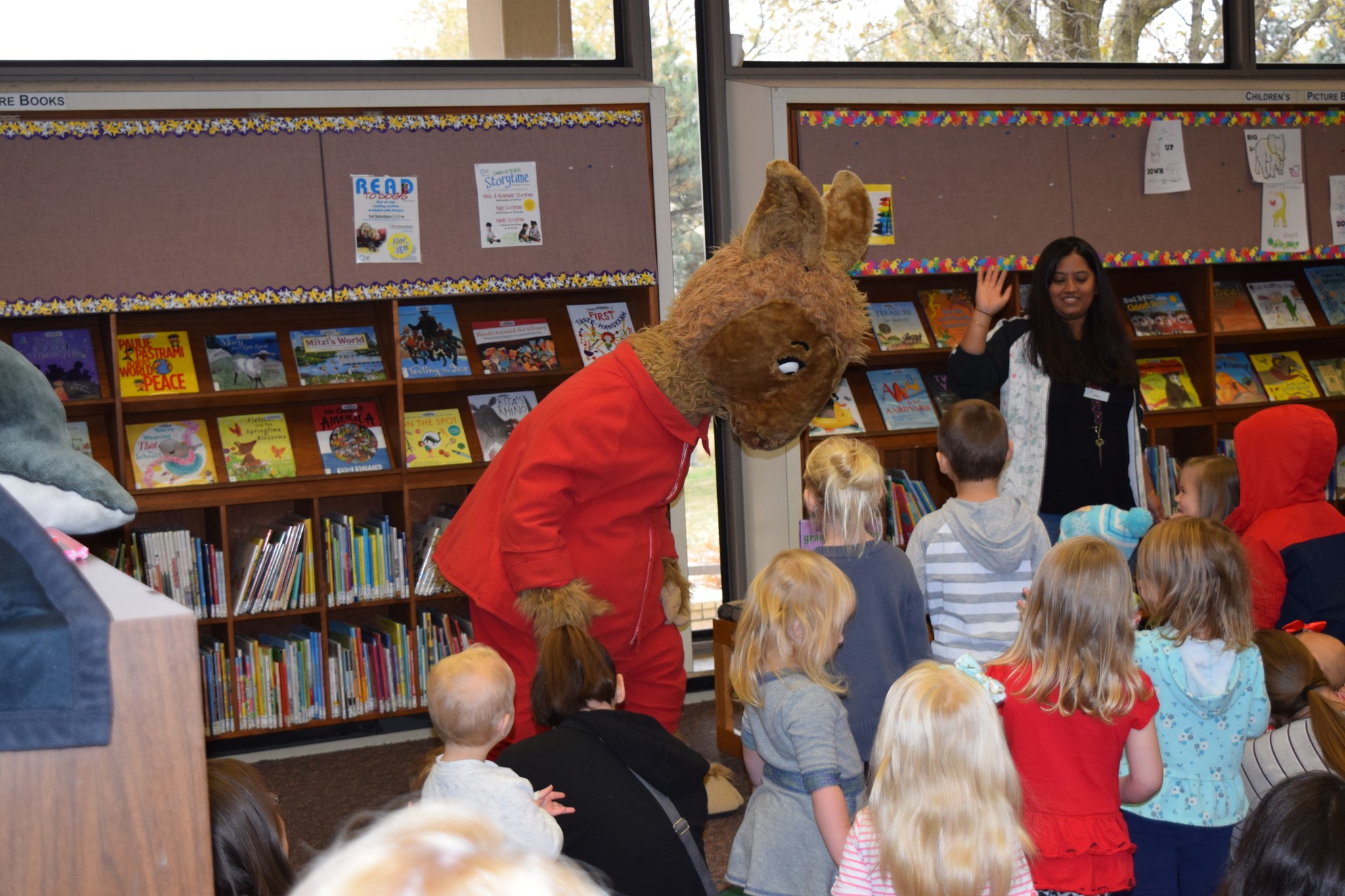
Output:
[430,162,881,767]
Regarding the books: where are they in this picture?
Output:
[18,263,1345,740]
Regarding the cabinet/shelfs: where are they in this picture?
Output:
[0,286,660,749]
[802,256,1345,644]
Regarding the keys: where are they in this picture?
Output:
[1095,438,1104,468]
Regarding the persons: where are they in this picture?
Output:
[951,237,1165,553]
[208,405,1345,896]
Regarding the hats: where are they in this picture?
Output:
[1057,504,1153,562]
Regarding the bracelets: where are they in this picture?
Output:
[975,308,992,317]
[1146,489,1155,500]
[973,318,991,329]
